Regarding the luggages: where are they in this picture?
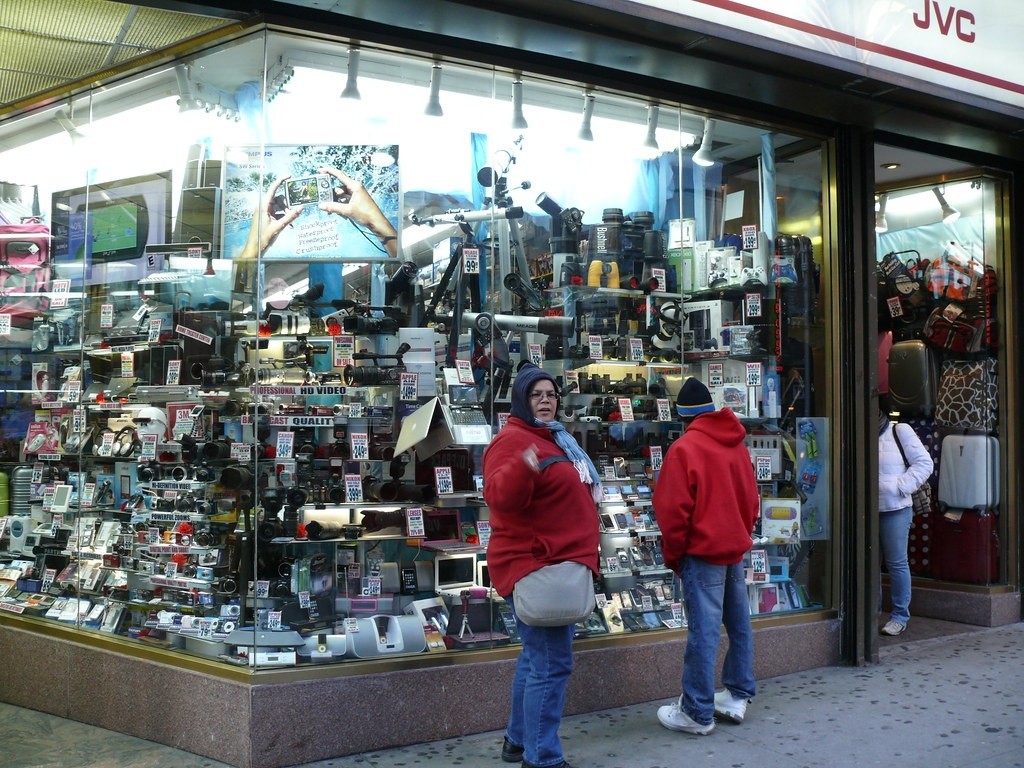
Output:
[878,328,1003,585]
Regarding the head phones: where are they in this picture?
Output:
[92,427,114,455]
[112,425,136,457]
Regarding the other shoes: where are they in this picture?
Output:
[501,736,525,762]
[521,758,573,768]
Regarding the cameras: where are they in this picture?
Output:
[285,173,333,209]
[124,207,677,637]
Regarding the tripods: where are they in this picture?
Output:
[459,597,475,639]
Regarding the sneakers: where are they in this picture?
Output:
[714,688,752,723]
[881,620,907,635]
[656,693,716,735]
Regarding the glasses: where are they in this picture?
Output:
[528,391,559,400]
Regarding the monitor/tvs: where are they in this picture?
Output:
[52,170,172,292]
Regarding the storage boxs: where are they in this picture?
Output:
[749,498,801,615]
[679,300,736,353]
[415,237,464,287]
[355,328,521,397]
[45,597,106,630]
[403,597,450,633]
[668,248,742,294]
[135,385,201,402]
[18,578,42,593]
[497,613,518,643]
[300,507,402,536]
[702,357,782,418]
[96,463,143,509]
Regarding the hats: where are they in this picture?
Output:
[676,377,715,418]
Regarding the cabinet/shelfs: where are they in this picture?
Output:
[0,283,786,643]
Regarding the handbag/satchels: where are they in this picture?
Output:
[513,561,596,628]
[877,249,998,355]
[892,423,932,515]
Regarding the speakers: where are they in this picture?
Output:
[204,160,222,187]
[187,160,200,188]
[178,184,221,260]
[345,613,427,659]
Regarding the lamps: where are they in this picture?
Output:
[511,79,527,129]
[875,192,887,233]
[425,64,444,116]
[642,105,659,150]
[933,184,960,223]
[177,82,241,122]
[692,116,715,166]
[339,49,361,100]
[174,61,199,114]
[577,92,595,141]
[55,99,85,141]
[260,66,294,102]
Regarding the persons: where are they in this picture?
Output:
[879,406,934,636]
[239,167,398,259]
[482,359,604,768]
[652,377,759,736]
